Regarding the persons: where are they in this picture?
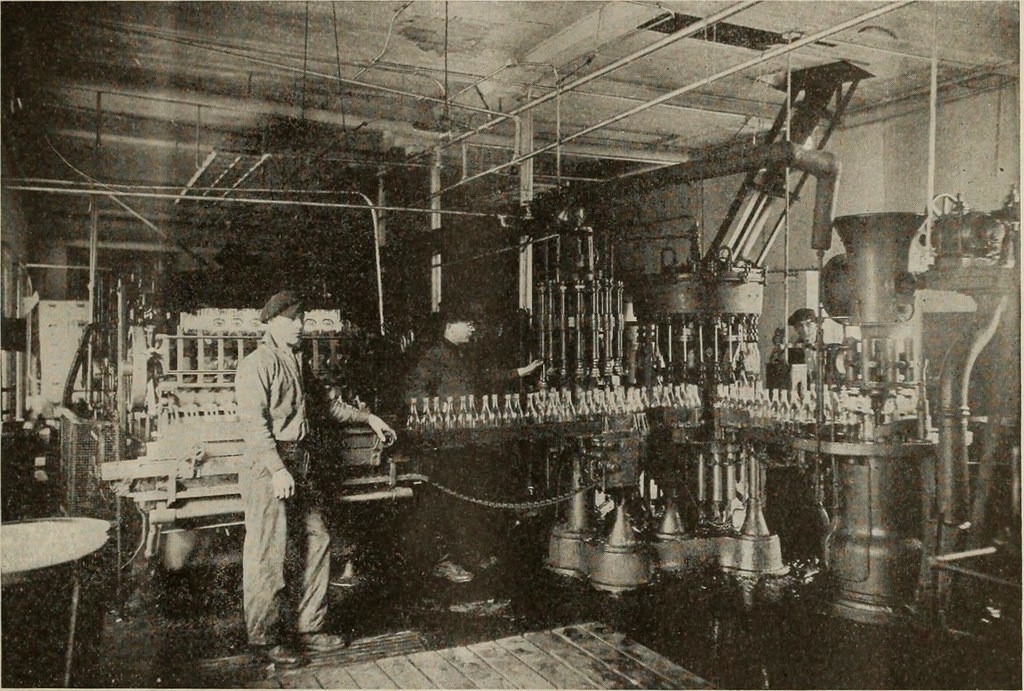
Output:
[400,303,545,584]
[770,309,820,384]
[234,289,397,669]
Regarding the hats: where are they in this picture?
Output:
[789,309,816,326]
[260,290,302,325]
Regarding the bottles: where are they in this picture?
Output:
[407,380,698,427]
[714,380,918,430]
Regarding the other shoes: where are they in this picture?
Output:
[252,644,307,669]
[286,633,347,652]
